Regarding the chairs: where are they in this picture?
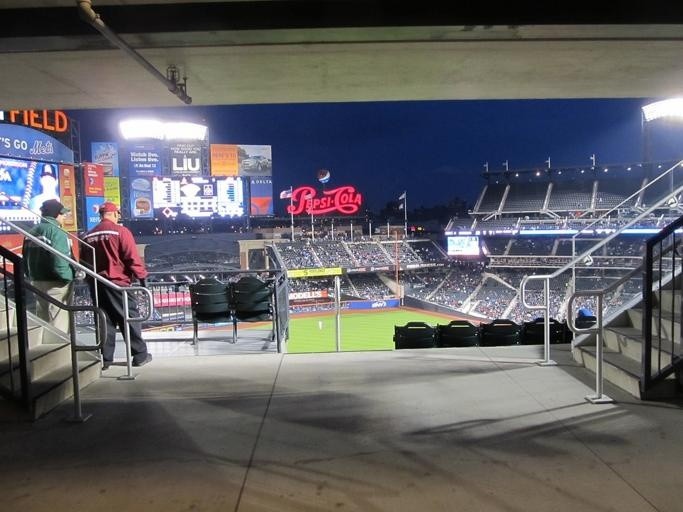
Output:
[392,316,596,349]
[189,278,237,345]
[231,277,276,341]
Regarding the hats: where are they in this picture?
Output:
[58,205,71,216]
[97,201,121,215]
[578,307,592,321]
[39,163,56,180]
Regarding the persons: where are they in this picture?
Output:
[77,201,153,370]
[21,198,77,333]
[318,320,323,330]
[266,227,447,306]
[447,197,683,323]
[29,164,60,208]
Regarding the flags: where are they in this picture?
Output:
[279,189,293,200]
[399,203,404,210]
[398,193,406,200]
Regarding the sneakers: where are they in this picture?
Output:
[131,350,153,367]
